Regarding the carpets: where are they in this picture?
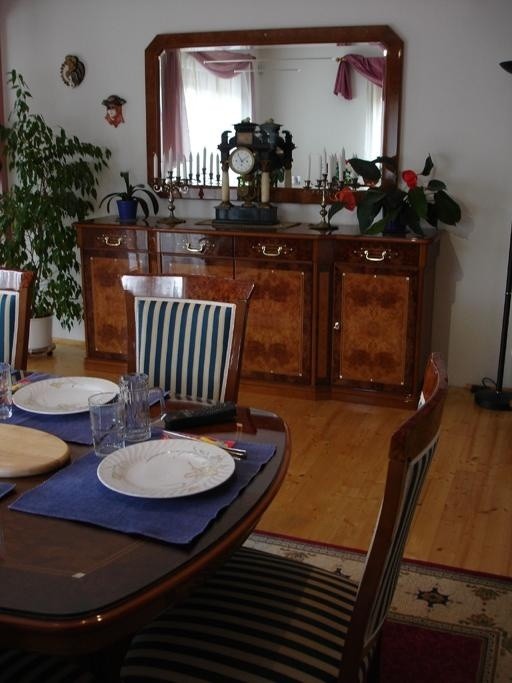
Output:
[242,529,512,683]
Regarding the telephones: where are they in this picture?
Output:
[163,399,236,428]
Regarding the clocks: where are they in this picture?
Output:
[229,147,255,175]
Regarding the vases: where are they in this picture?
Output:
[328,153,461,234]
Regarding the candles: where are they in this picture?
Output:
[318,154,322,181]
[338,153,343,181]
[322,146,327,174]
[183,154,187,179]
[353,152,357,178]
[161,152,166,179]
[328,153,332,182]
[197,152,200,175]
[306,153,311,180]
[210,152,213,173]
[177,153,180,177]
[342,147,345,172]
[216,153,220,175]
[168,145,173,170]
[203,147,206,168]
[189,152,192,174]
[153,151,159,178]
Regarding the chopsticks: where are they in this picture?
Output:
[161,426,248,461]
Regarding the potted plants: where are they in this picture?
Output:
[1,70,112,356]
[99,171,158,220]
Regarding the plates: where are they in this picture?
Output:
[10,374,119,416]
[96,437,236,500]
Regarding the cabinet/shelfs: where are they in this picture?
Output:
[151,217,234,278]
[72,215,152,362]
[236,222,329,390]
[325,228,445,404]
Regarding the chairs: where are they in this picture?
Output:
[118,353,448,683]
[0,269,33,374]
[119,275,256,403]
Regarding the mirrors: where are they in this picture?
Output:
[144,25,405,205]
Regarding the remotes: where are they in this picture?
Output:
[163,402,237,431]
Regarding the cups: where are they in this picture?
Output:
[118,374,169,443]
[0,363,16,422]
[88,389,127,458]
